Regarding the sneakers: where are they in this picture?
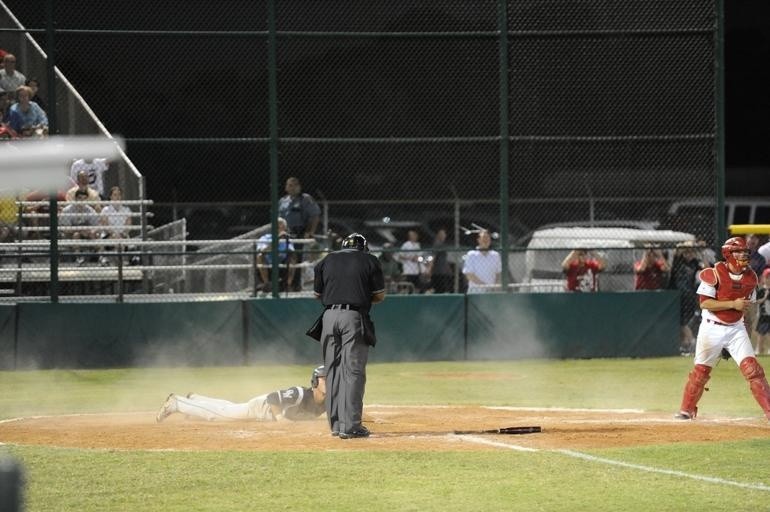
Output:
[333,425,369,438]
[157,393,175,420]
[675,411,691,421]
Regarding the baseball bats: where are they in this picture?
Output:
[454,426,542,435]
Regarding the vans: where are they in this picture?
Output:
[304,189,770,294]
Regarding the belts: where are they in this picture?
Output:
[325,304,359,311]
[707,319,729,326]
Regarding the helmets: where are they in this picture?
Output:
[342,233,366,250]
[722,237,751,270]
[311,366,326,388]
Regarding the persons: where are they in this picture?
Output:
[0,46,142,267]
[306,232,387,440]
[158,364,326,425]
[673,236,770,420]
[562,231,769,358]
[247,177,501,294]
